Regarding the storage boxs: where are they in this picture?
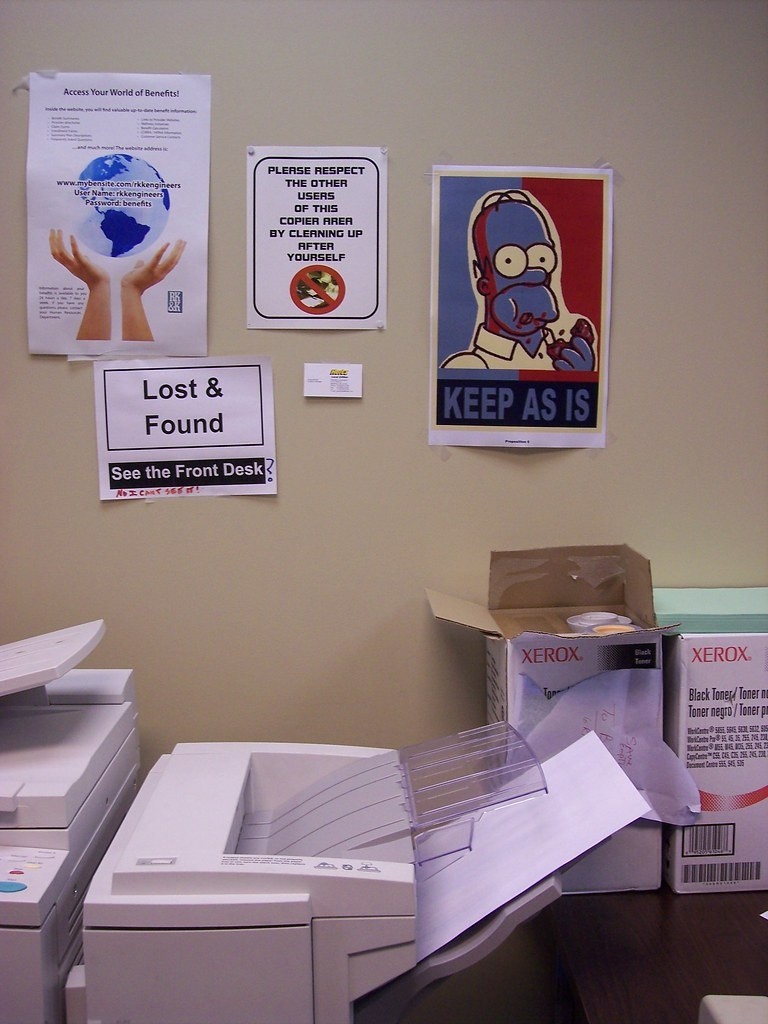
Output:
[423,543,683,894]
[663,633,768,894]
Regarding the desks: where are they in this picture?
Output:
[550,891,767,1024]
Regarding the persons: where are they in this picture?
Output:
[49,227,187,342]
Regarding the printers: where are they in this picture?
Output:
[0,621,650,1024]
